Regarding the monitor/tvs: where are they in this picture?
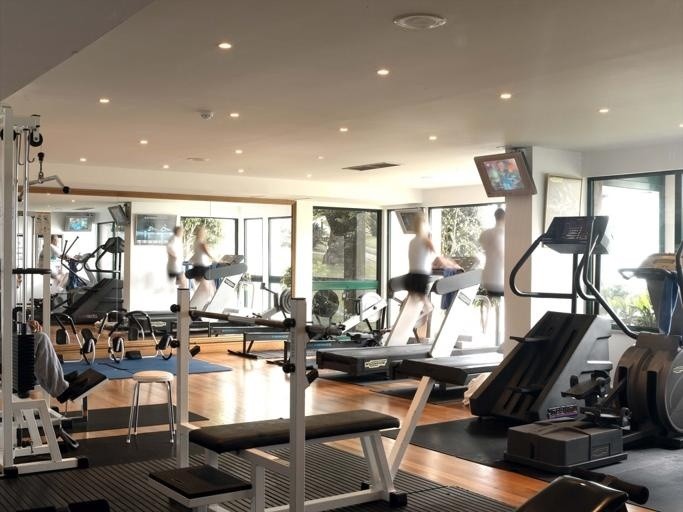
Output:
[474,153,538,198]
[135,214,177,245]
[107,205,131,226]
[64,216,92,231]
[326,210,357,235]
[395,209,423,234]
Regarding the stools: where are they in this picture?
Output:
[126,370,175,443]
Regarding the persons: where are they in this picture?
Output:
[478,208,503,340]
[189,227,220,307]
[406,223,460,346]
[165,228,187,289]
[39,234,70,292]
[26,320,88,403]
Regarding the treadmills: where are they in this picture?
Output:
[132,254,247,331]
[315,267,507,387]
